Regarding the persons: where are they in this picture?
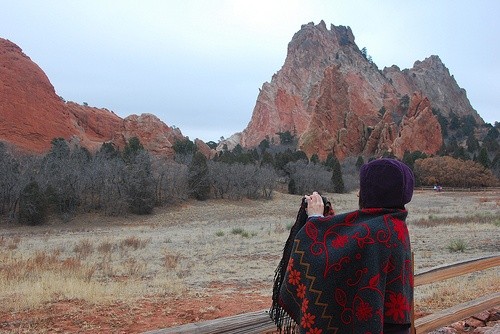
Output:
[433,185,443,192]
[268,157,414,334]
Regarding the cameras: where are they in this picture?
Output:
[304,199,307,208]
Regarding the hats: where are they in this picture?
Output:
[359,157,414,208]
